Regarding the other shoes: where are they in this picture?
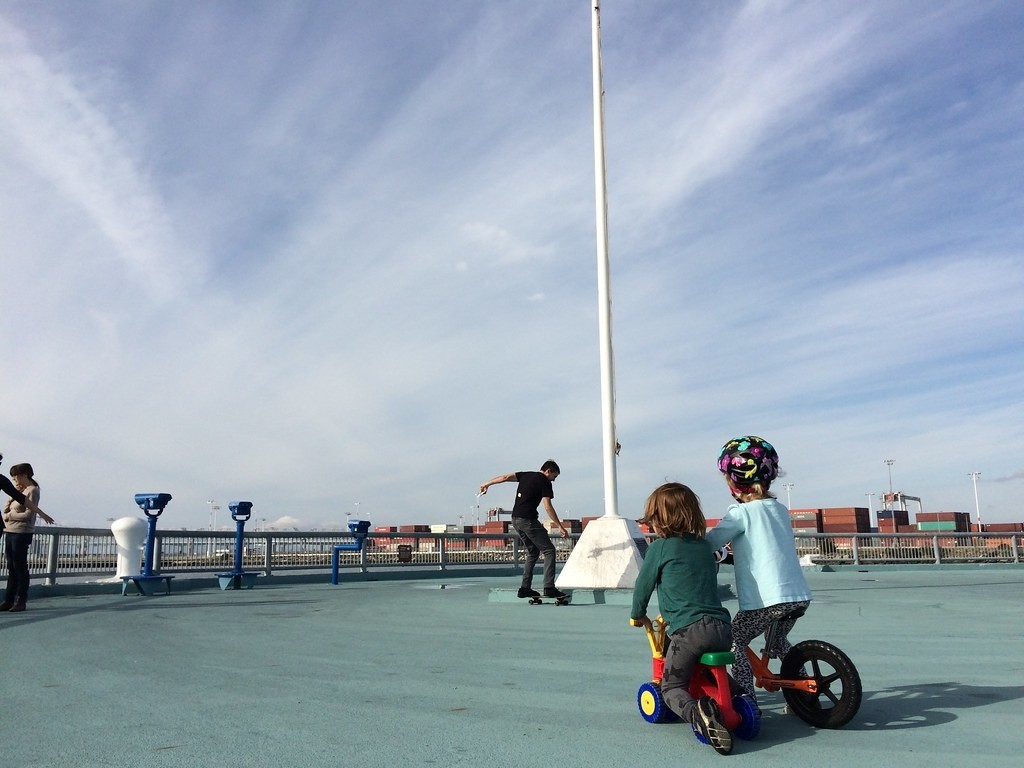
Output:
[544,587,566,596]
[784,698,822,715]
[517,587,540,598]
[9,597,26,611]
[0,600,15,611]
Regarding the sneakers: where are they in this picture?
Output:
[692,696,733,755]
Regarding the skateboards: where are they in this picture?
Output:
[527,593,570,606]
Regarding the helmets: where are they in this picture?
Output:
[717,436,779,484]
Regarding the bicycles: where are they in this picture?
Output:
[742,608,863,731]
[627,612,763,743]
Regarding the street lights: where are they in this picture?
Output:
[864,493,876,527]
[212,506,222,558]
[354,501,361,518]
[206,500,215,560]
[344,510,353,531]
[780,483,797,512]
[882,459,897,547]
[475,490,486,550]
[967,471,981,533]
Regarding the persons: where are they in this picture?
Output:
[631,483,733,756]
[705,435,810,715]
[480,461,568,598]
[0,463,40,612]
[0,454,55,540]
